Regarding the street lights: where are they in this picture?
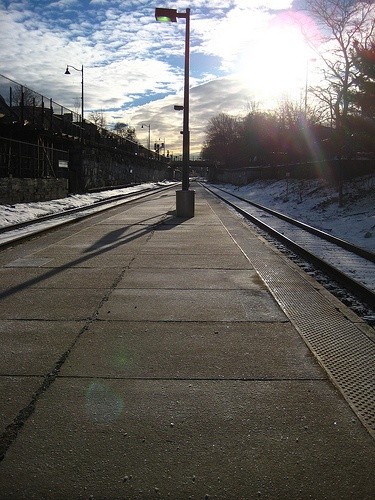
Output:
[141,124,151,151]
[154,6,194,220]
[64,65,84,129]
